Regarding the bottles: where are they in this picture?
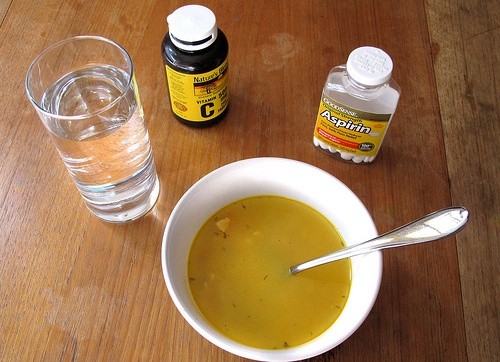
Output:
[313,46,401,165]
[160,4,231,129]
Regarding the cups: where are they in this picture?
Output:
[25,35,160,223]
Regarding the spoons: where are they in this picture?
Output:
[288,205,468,275]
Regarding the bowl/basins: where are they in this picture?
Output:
[162,157,381,361]
